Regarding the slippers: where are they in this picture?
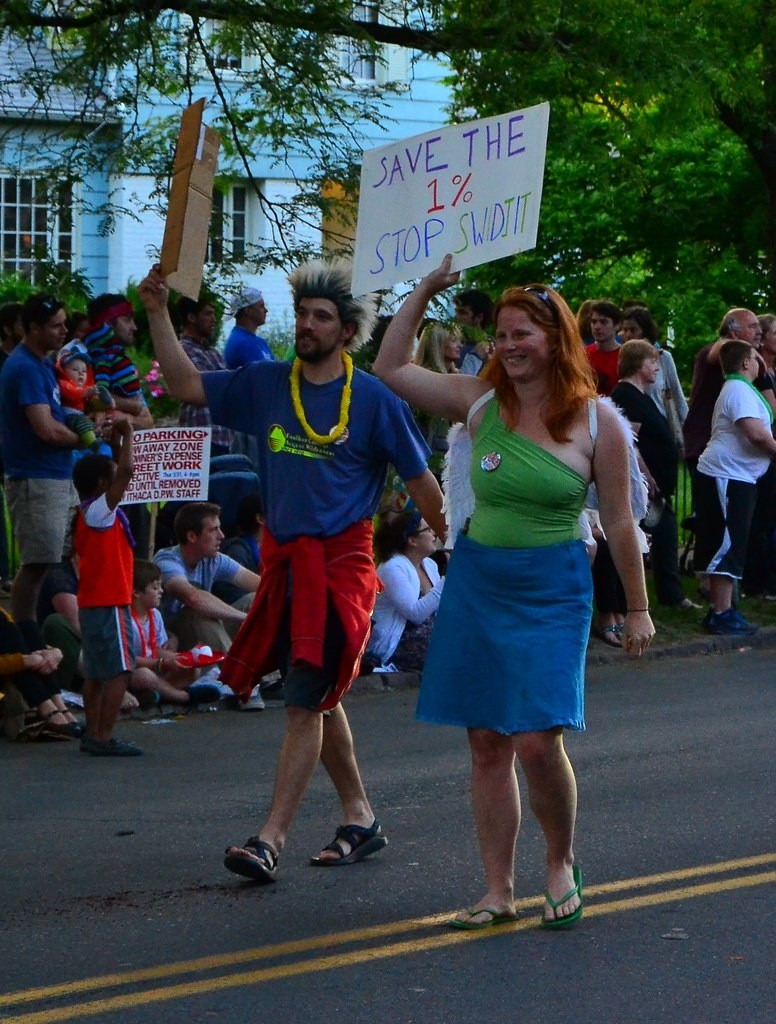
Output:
[450,909,520,930]
[542,864,584,929]
[589,617,624,648]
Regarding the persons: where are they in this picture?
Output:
[0,286,776,756]
[137,263,451,884]
[372,255,655,930]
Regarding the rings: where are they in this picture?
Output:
[107,419,112,425]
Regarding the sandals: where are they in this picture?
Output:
[225,836,278,884]
[312,819,388,866]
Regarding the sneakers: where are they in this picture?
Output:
[80,737,141,757]
[258,673,282,692]
[239,686,264,711]
[703,606,759,636]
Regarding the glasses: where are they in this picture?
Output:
[522,287,559,322]
[42,296,59,312]
[416,527,431,534]
[748,356,760,363]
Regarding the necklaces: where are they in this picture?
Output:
[290,352,354,445]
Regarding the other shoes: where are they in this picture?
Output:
[39,709,84,741]
[180,685,220,707]
[679,598,702,608]
[128,688,160,710]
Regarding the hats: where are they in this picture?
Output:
[224,289,262,321]
[61,347,92,365]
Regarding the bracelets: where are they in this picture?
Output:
[157,658,166,675]
[154,659,160,675]
[627,607,650,612]
